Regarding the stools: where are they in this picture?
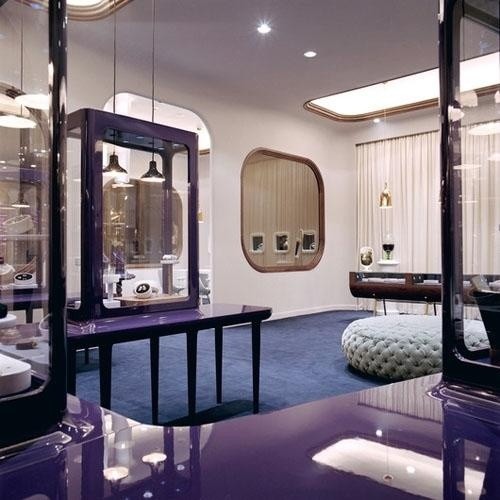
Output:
[341,314,490,380]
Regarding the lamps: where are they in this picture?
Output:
[0,0,173,186]
[376,117,393,209]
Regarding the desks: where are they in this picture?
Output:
[67,301,273,425]
[0,369,500,500]
